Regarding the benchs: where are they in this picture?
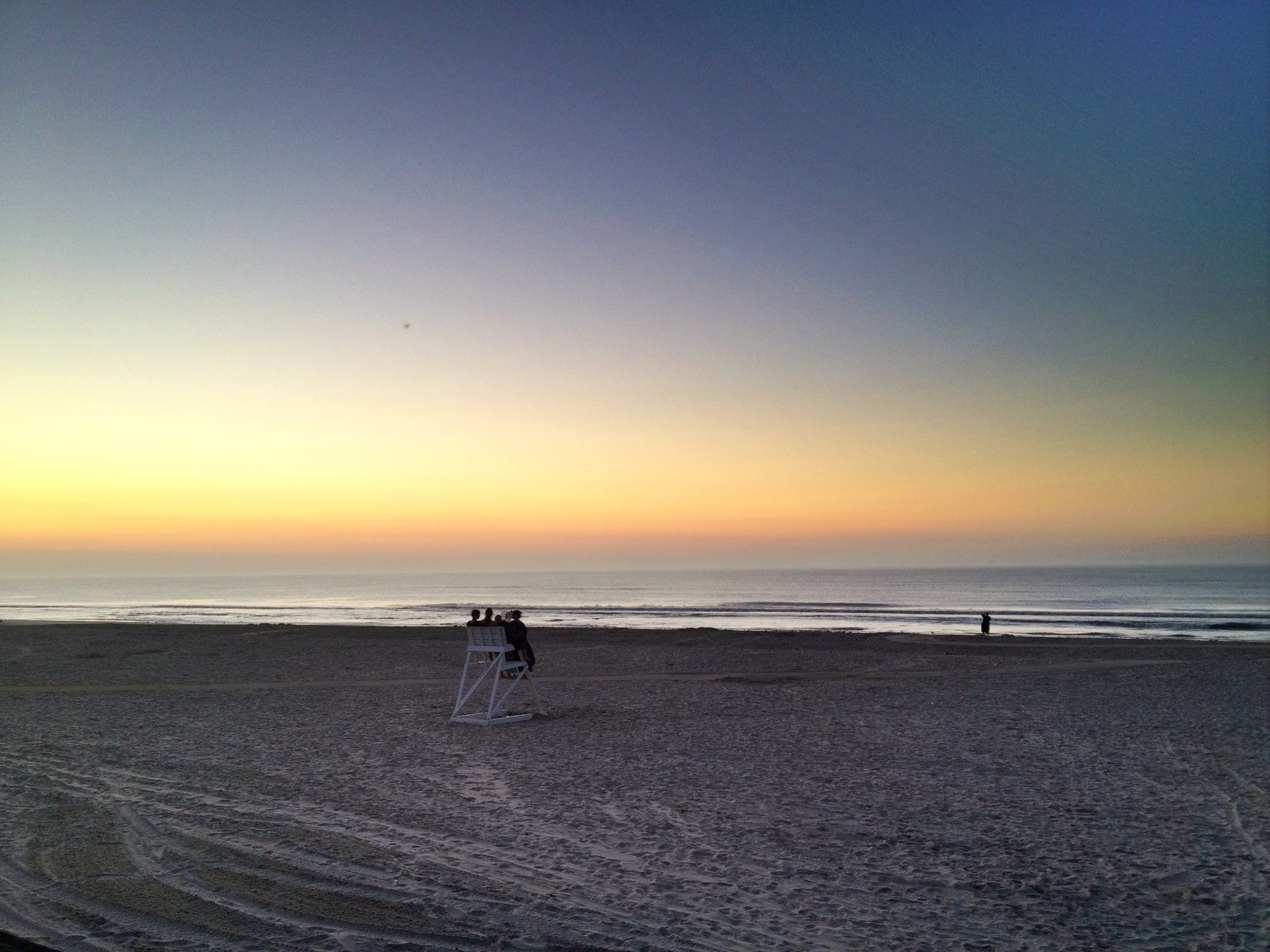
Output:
[447,626,547,727]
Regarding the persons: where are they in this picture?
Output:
[981,611,991,636]
[465,607,536,680]
